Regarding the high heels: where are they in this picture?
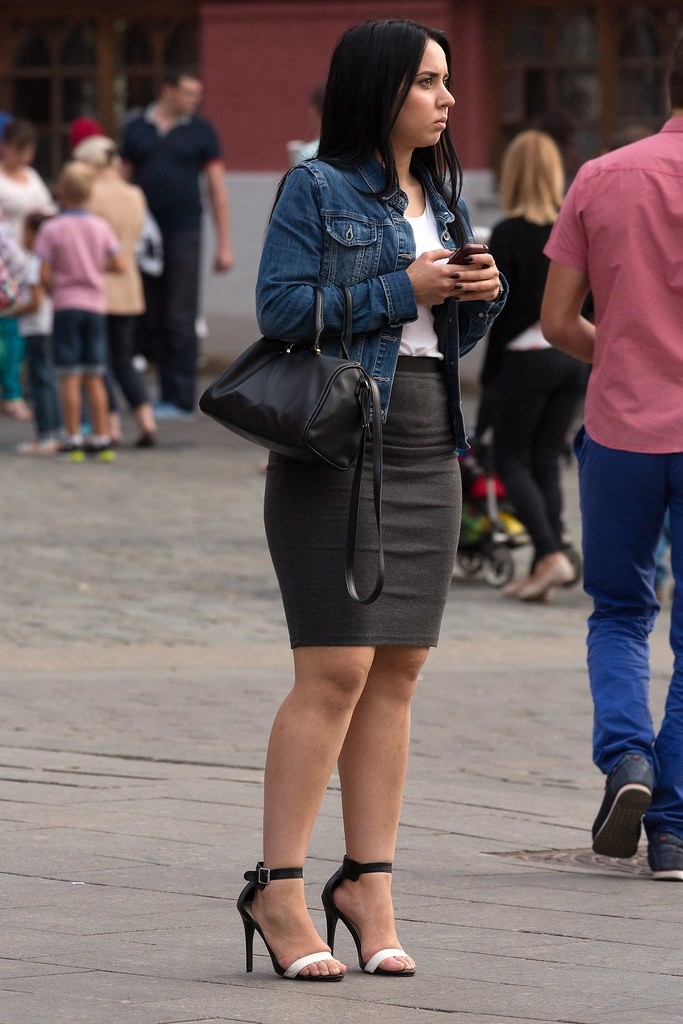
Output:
[322,855,416,976]
[236,862,345,982]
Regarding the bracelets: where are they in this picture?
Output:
[496,281,503,303]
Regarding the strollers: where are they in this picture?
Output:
[452,420,534,590]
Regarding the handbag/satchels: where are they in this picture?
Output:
[199,287,373,472]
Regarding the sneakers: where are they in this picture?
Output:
[646,835,683,880]
[591,754,653,859]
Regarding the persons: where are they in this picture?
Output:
[0,69,232,463]
[481,37,683,883]
[234,18,510,982]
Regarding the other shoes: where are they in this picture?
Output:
[135,430,157,450]
[52,444,84,464]
[17,436,61,458]
[500,554,576,600]
[153,399,194,424]
[2,399,32,421]
[517,554,576,603]
[84,444,116,463]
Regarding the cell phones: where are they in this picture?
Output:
[447,244,489,264]
